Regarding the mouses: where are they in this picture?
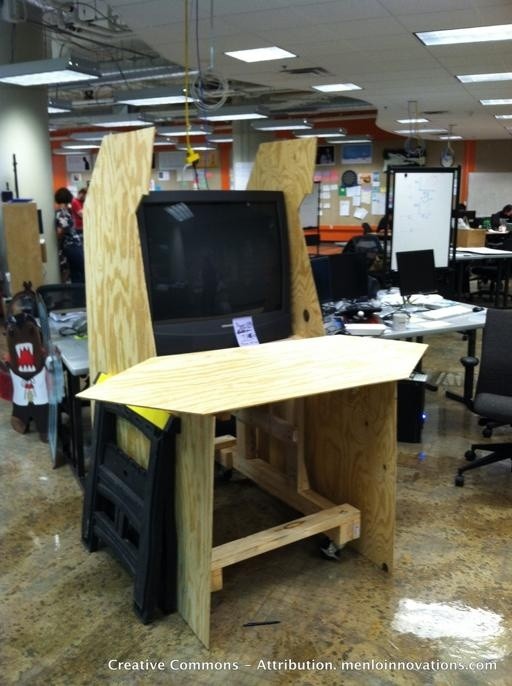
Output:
[473,307,484,313]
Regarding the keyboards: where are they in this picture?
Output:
[421,305,472,321]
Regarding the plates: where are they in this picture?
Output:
[343,323,385,336]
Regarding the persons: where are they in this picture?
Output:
[450,208,472,229]
[454,203,470,227]
[54,186,84,284]
[72,187,86,234]
[375,207,393,233]
[491,204,512,230]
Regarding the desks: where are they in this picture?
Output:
[36,316,90,494]
[370,248,512,298]
[380,299,487,409]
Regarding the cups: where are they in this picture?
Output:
[392,313,409,330]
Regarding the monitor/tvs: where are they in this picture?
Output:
[137,190,292,357]
[396,249,438,297]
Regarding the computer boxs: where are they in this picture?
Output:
[397,373,428,444]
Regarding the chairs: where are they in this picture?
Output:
[455,308,512,488]
[472,231,512,309]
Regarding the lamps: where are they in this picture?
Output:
[0,55,374,156]
